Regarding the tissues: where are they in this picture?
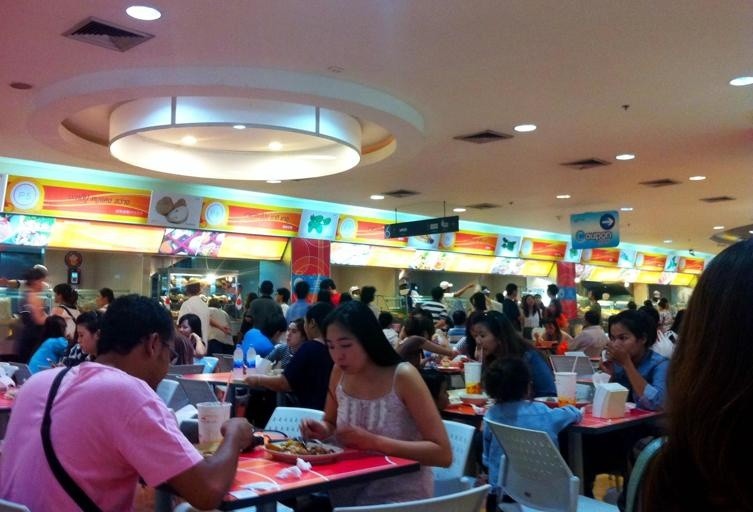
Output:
[590,372,629,418]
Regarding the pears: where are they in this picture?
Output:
[157,197,188,223]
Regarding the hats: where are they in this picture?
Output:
[440,281,453,290]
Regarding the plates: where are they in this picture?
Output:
[534,396,591,408]
[263,439,345,464]
[459,395,489,406]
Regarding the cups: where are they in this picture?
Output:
[463,362,483,394]
[196,400,232,444]
[555,370,577,410]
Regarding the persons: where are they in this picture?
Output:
[0,263,687,512]
[654,233,753,512]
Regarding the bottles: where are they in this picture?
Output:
[233,344,244,379]
[246,343,258,376]
